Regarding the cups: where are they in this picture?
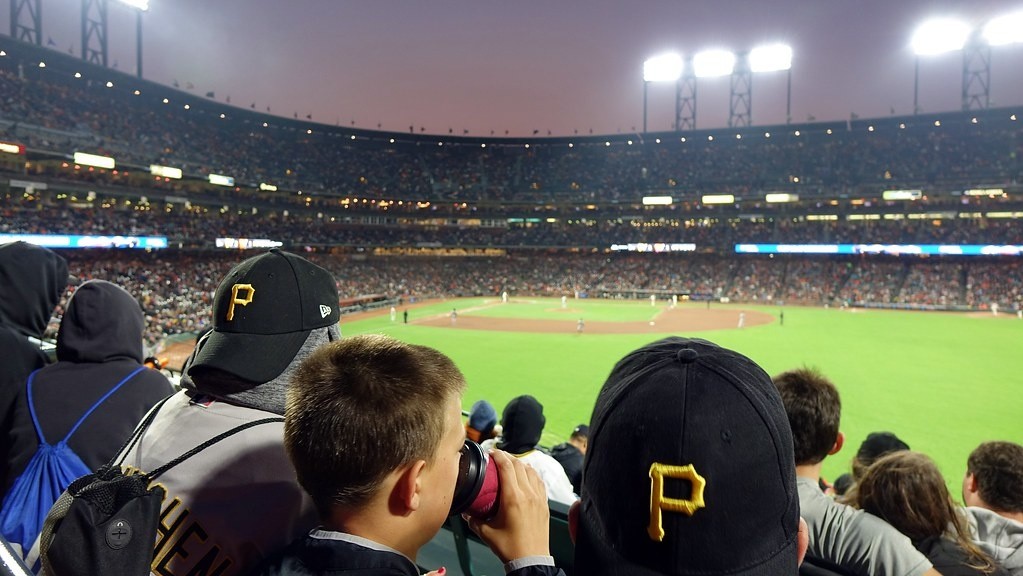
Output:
[448,438,501,520]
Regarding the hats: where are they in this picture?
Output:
[188,248,342,382]
[576,336,801,576]
[467,400,497,441]
[857,431,910,464]
[574,424,588,436]
[833,474,852,494]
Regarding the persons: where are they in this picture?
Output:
[672,295,677,306]
[990,302,998,315]
[450,308,457,327]
[650,294,656,304]
[780,312,783,326]
[560,295,567,307]
[738,311,745,327]
[390,305,396,320]
[576,318,584,333]
[0,89,1023,576]
[403,308,408,324]
[502,291,508,302]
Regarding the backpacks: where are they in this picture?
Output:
[39,393,286,576]
[0,366,147,573]
